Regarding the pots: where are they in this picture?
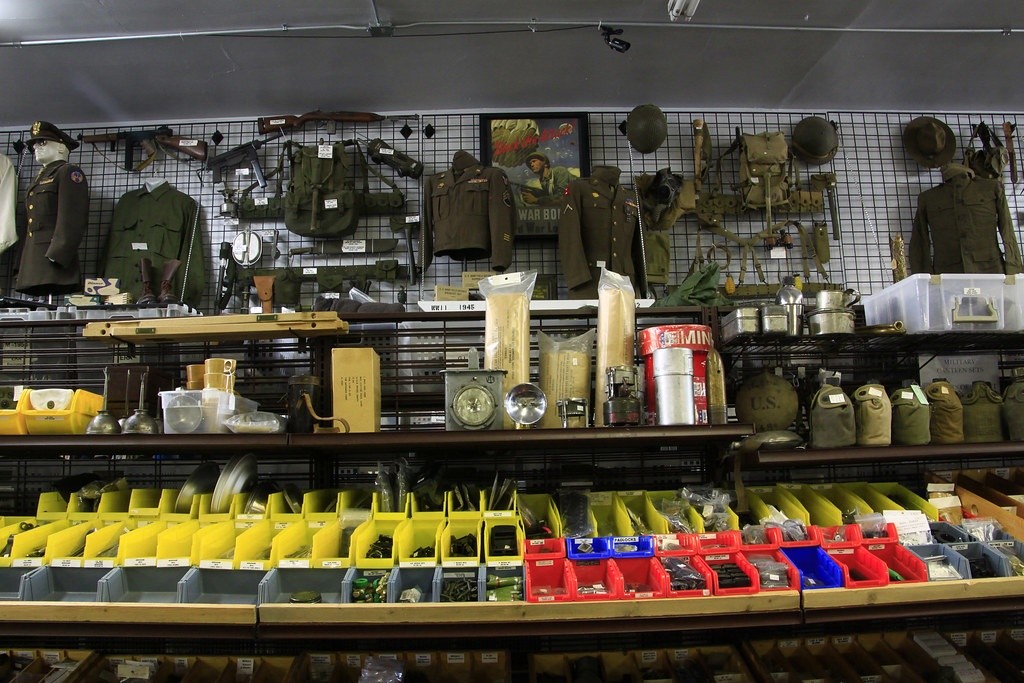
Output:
[805,287,862,334]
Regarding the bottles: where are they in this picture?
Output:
[776,275,804,336]
[85,409,164,434]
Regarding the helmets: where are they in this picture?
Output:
[525,152,549,168]
[792,117,839,165]
[627,105,667,154]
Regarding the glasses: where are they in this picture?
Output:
[30,139,47,150]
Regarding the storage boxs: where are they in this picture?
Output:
[1003,273,1024,330]
[862,273,1006,332]
[158,391,261,434]
[0,604,1024,683]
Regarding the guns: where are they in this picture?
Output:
[509,180,543,197]
[206,128,292,189]
[81,126,209,171]
[257,106,420,136]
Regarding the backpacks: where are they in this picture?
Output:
[278,138,368,237]
[717,132,801,208]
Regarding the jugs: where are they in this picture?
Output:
[808,366,1024,446]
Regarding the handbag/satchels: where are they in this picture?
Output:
[965,124,1009,179]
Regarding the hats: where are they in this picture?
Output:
[903,117,956,169]
[25,121,79,150]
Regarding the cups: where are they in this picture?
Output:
[165,358,236,433]
[652,347,694,425]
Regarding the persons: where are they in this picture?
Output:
[98,176,208,305]
[14,121,89,296]
[522,152,580,205]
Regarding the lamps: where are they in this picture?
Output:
[668,0,700,23]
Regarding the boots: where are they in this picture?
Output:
[138,259,156,303]
[158,259,182,303]
